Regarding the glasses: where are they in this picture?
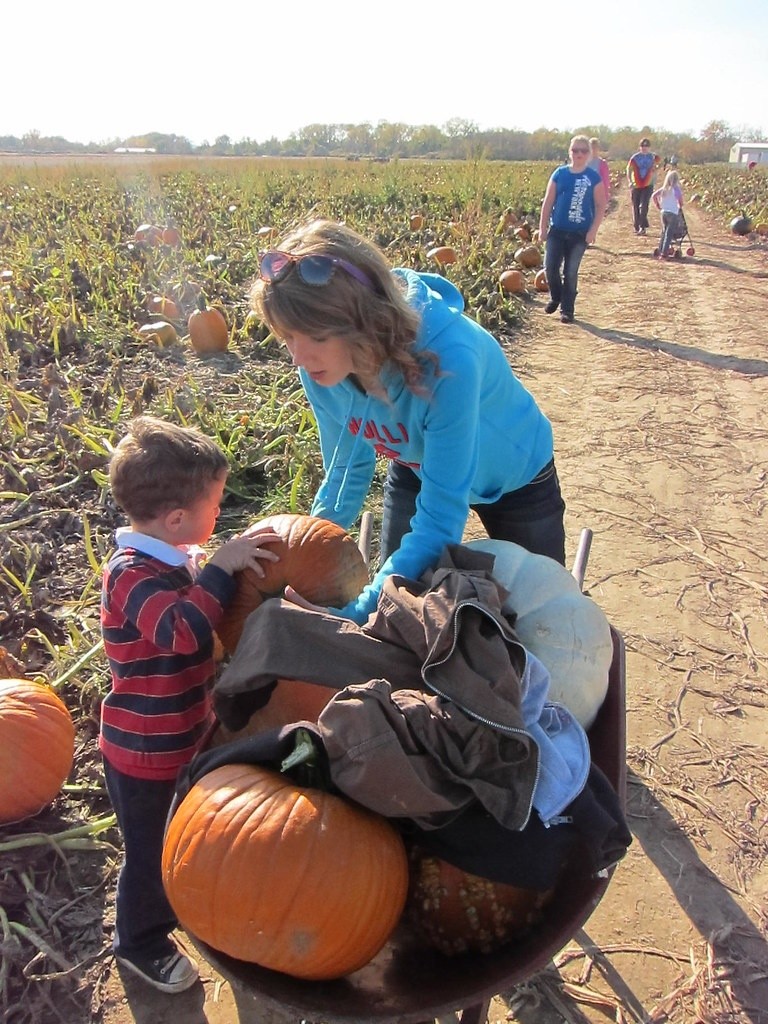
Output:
[640,143,650,148]
[258,249,379,292]
[569,148,590,153]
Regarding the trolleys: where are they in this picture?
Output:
[172,523,630,1024]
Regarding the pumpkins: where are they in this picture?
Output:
[393,857,557,968]
[211,514,370,656]
[0,677,74,824]
[411,212,551,294]
[160,729,409,980]
[130,226,278,356]
[455,539,614,734]
[203,679,339,751]
[730,209,768,236]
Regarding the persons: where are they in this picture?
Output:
[749,161,757,169]
[627,139,661,236]
[663,153,679,168]
[539,135,609,323]
[250,221,567,627]
[98,417,283,992]
[652,171,684,261]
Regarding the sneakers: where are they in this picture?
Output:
[114,938,199,994]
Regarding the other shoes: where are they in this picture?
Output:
[544,297,559,314]
[633,224,638,232]
[639,228,647,236]
[560,303,575,323]
[656,252,671,260]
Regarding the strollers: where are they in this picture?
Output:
[652,206,695,259]
